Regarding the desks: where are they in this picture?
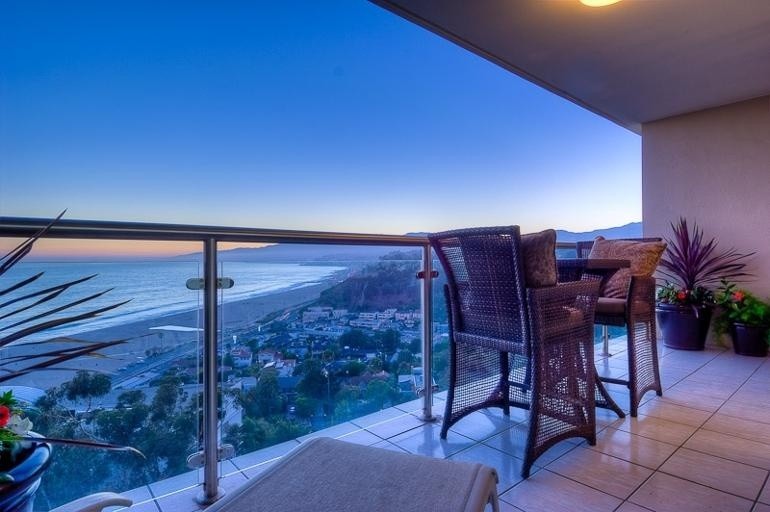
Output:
[479,257,631,421]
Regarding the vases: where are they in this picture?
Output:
[654,300,714,351]
[729,321,770,356]
[0,430,53,512]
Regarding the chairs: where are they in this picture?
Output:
[42,435,502,511]
[427,226,626,480]
[574,238,663,416]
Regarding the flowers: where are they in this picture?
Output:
[709,282,770,348]
[0,209,149,458]
[656,215,758,305]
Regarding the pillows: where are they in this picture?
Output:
[518,229,559,289]
[576,236,669,298]
